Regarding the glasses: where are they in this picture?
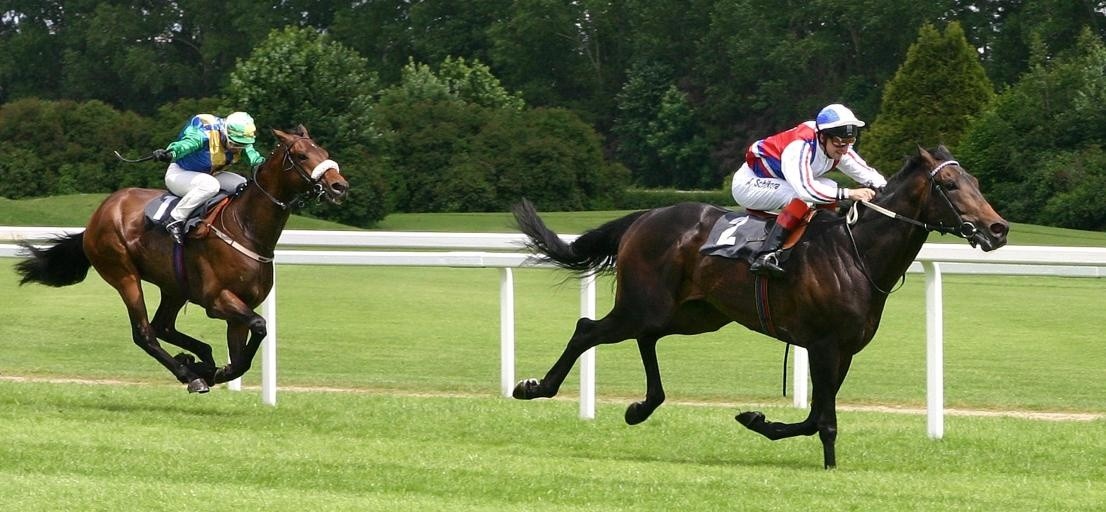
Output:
[829,136,858,148]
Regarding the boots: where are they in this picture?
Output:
[748,222,790,281]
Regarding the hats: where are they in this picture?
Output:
[223,112,258,147]
[815,102,867,136]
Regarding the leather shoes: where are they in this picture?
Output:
[161,215,184,246]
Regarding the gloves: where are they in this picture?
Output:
[152,149,173,163]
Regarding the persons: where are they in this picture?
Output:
[150,110,266,246]
[729,103,887,281]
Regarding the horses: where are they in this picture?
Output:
[10,123,351,393]
[506,142,1010,469]
